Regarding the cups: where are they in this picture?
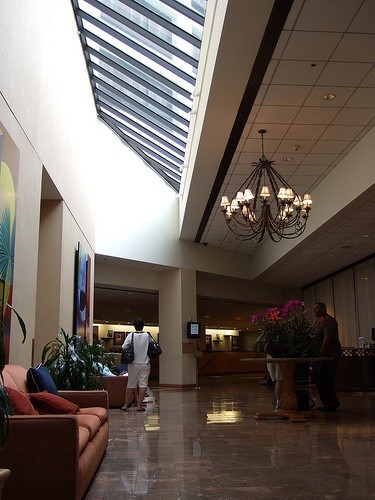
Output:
[364,341,370,348]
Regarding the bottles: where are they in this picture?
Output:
[358,336,363,349]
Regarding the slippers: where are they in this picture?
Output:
[137,408,145,411]
[121,407,127,411]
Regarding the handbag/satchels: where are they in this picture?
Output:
[121,333,134,365]
[148,332,162,358]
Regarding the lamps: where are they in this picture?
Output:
[219,129,313,244]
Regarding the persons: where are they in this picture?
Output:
[121,320,154,412]
[267,353,283,409]
[92,353,149,407]
[311,303,342,412]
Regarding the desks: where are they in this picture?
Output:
[239,356,335,424]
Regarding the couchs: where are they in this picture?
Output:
[81,360,138,407]
[0,362,110,500]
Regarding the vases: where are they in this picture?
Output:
[260,336,312,358]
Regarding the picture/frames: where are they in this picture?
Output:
[93,326,98,345]
[113,331,131,346]
[73,241,92,352]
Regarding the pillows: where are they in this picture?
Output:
[26,362,59,394]
[29,393,81,416]
[3,386,40,416]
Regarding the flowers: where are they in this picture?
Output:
[248,299,320,352]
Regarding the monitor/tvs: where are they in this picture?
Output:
[187,322,202,338]
